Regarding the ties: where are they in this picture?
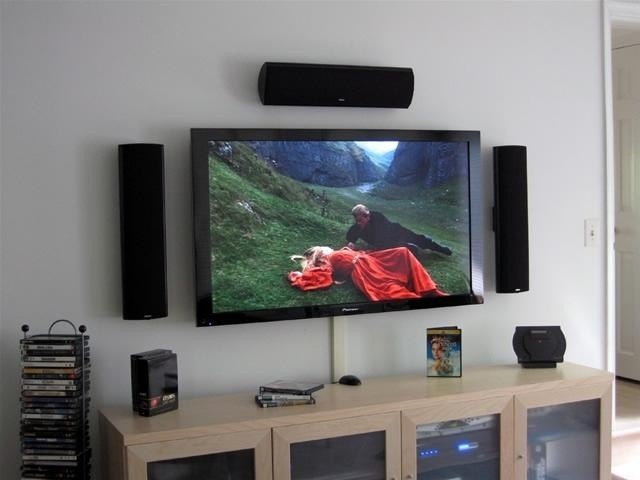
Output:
[189,127,485,327]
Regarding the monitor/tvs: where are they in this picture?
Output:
[255,379,324,408]
[19,334,91,480]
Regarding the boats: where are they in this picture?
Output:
[492,145,530,294]
[257,61,414,109]
[118,142,169,321]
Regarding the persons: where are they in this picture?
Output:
[288,204,452,301]
[430,335,453,375]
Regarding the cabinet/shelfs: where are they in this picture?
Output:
[96,360,613,480]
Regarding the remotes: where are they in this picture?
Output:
[338,374,363,386]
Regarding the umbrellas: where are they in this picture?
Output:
[432,290,451,297]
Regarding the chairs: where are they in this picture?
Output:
[438,244,452,255]
